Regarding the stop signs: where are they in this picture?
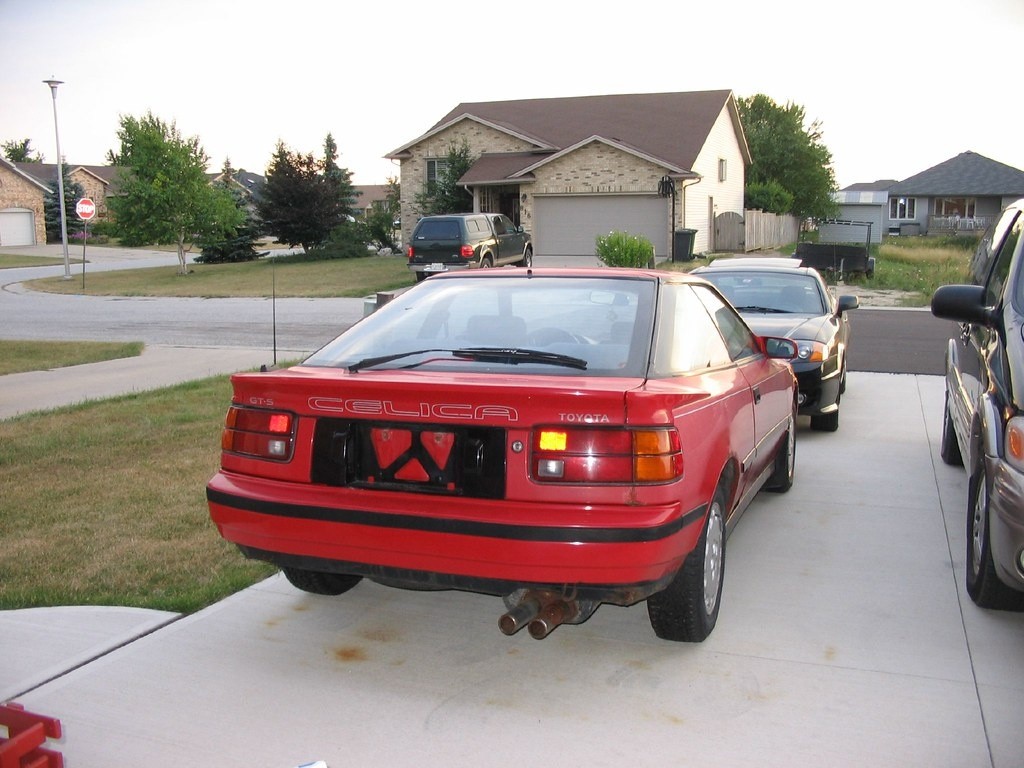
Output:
[76,199,95,219]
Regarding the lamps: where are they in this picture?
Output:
[719,158,727,182]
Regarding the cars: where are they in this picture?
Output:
[683,257,860,432]
[206,268,799,643]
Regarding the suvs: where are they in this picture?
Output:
[930,198,1024,610]
[406,213,534,282]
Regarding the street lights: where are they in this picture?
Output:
[42,74,72,281]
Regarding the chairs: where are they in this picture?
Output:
[467,314,527,345]
[716,285,744,306]
[598,320,634,345]
[767,286,816,312]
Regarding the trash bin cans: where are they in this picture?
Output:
[673,228,698,261]
